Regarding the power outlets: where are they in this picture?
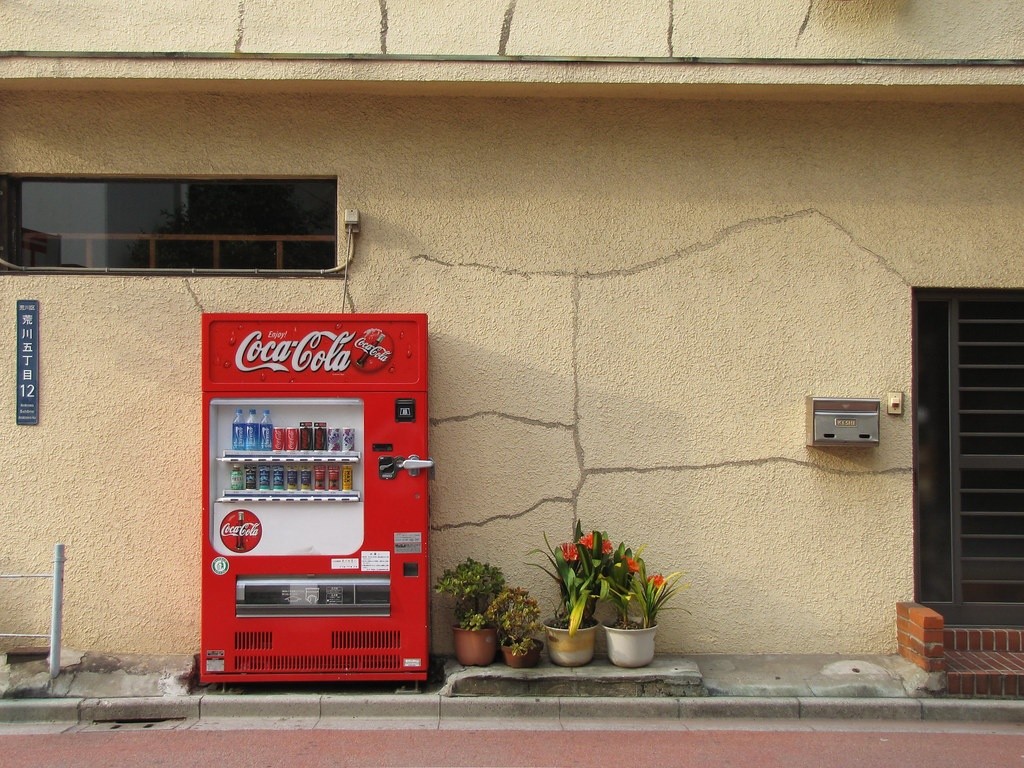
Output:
[344,209,360,234]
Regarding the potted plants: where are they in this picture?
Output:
[483,586,544,669]
[433,557,504,666]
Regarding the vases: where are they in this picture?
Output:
[601,617,659,667]
[541,612,600,667]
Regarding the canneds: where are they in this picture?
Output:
[299,421,327,451]
[326,427,355,451]
[244,464,354,492]
[272,427,299,451]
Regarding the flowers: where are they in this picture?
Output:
[524,519,692,637]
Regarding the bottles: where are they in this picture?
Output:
[234,512,245,550]
[356,334,385,367]
[259,410,273,450]
[245,464,353,492]
[230,465,243,490]
[298,422,355,452]
[231,408,246,450]
[245,409,259,451]
